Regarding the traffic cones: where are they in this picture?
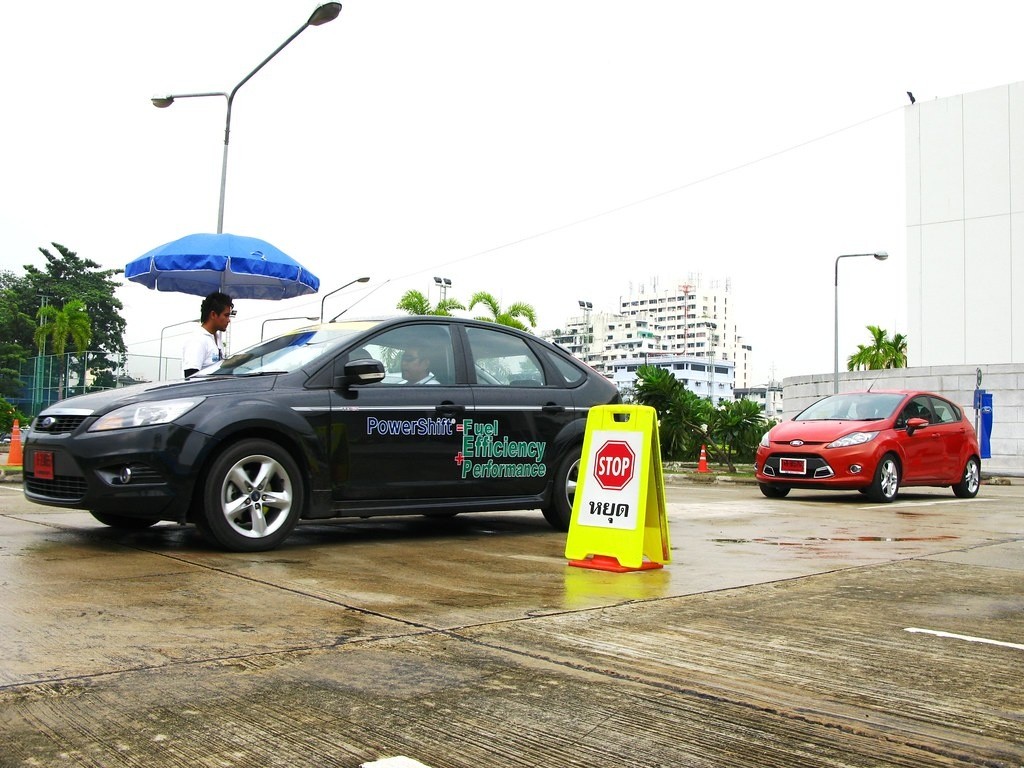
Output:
[0,419,24,467]
[692,444,716,474]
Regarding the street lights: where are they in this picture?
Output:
[225,310,238,355]
[261,316,320,341]
[159,318,202,381]
[742,344,752,388]
[579,300,593,364]
[151,1,343,234]
[321,277,370,324]
[833,250,889,395]
[433,276,452,302]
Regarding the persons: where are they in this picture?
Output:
[183,291,234,378]
[397,341,440,385]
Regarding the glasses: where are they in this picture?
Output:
[402,354,420,363]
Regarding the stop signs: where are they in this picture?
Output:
[594,440,635,491]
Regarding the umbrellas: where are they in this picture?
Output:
[124,233,320,350]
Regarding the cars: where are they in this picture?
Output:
[754,390,981,504]
[21,314,627,553]
